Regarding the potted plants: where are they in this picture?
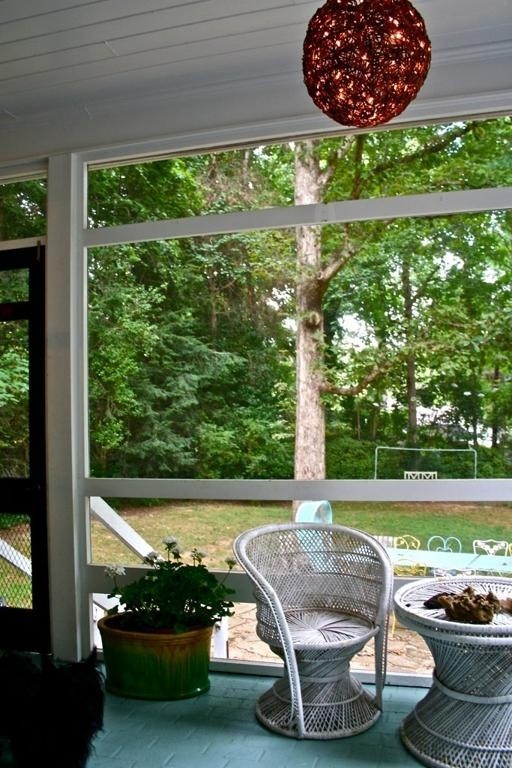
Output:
[97,536,238,700]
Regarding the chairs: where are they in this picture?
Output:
[232,523,395,739]
[375,536,512,576]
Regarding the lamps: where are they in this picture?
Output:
[301,1,432,129]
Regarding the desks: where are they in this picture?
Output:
[394,576,512,768]
